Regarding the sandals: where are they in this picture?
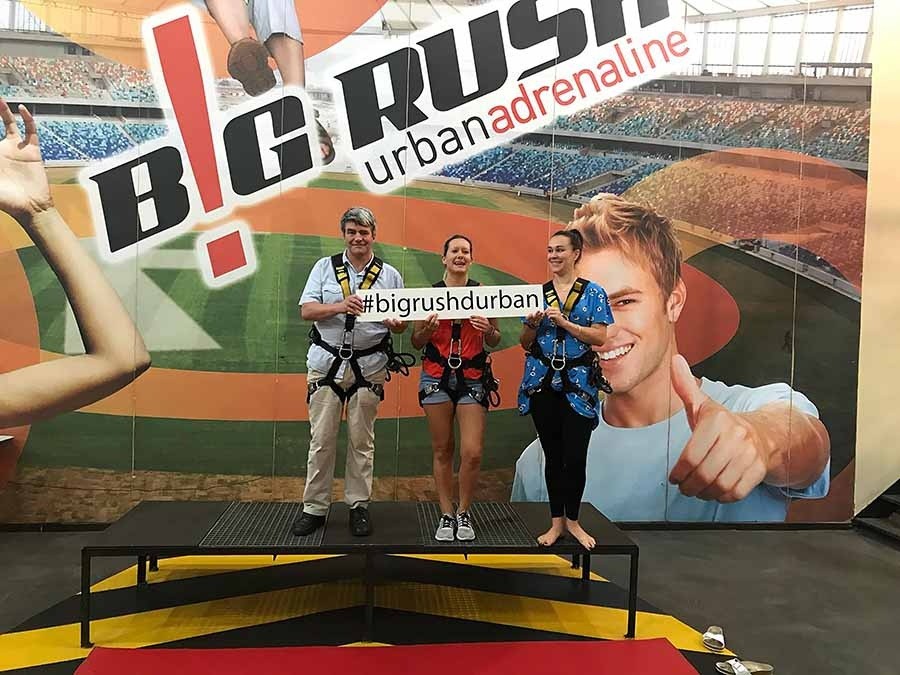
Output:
[229,39,277,96]
[313,109,335,165]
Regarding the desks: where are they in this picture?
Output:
[80,501,639,650]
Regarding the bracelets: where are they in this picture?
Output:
[489,326,495,337]
[524,320,536,329]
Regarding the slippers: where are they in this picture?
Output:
[715,657,774,675]
[703,626,726,651]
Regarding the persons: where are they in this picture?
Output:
[518,230,614,550]
[436,91,870,286]
[412,234,501,542]
[1,56,164,160]
[508,193,829,522]
[293,208,407,538]
[1,97,150,429]
[202,0,335,165]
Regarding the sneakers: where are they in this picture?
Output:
[350,507,367,535]
[435,512,455,541]
[455,509,475,540]
[293,513,325,535]
